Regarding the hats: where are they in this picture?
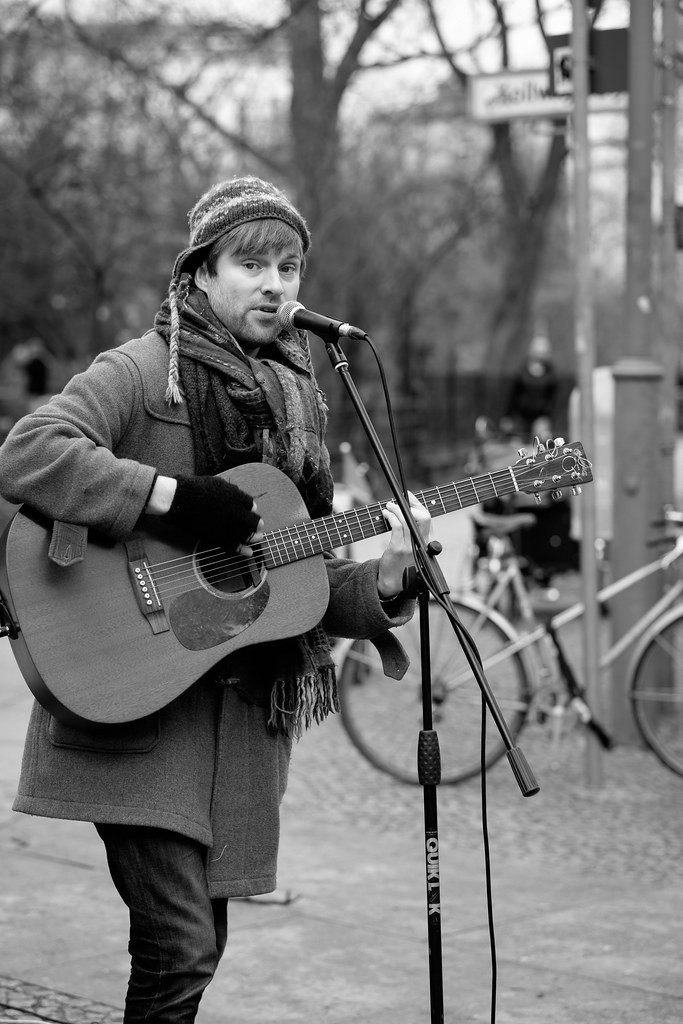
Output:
[163,176,330,416]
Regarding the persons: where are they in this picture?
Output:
[0,178,432,1024]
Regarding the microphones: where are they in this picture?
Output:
[277,300,369,341]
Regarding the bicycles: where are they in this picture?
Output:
[332,506,682,786]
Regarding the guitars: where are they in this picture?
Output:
[2,436,593,740]
